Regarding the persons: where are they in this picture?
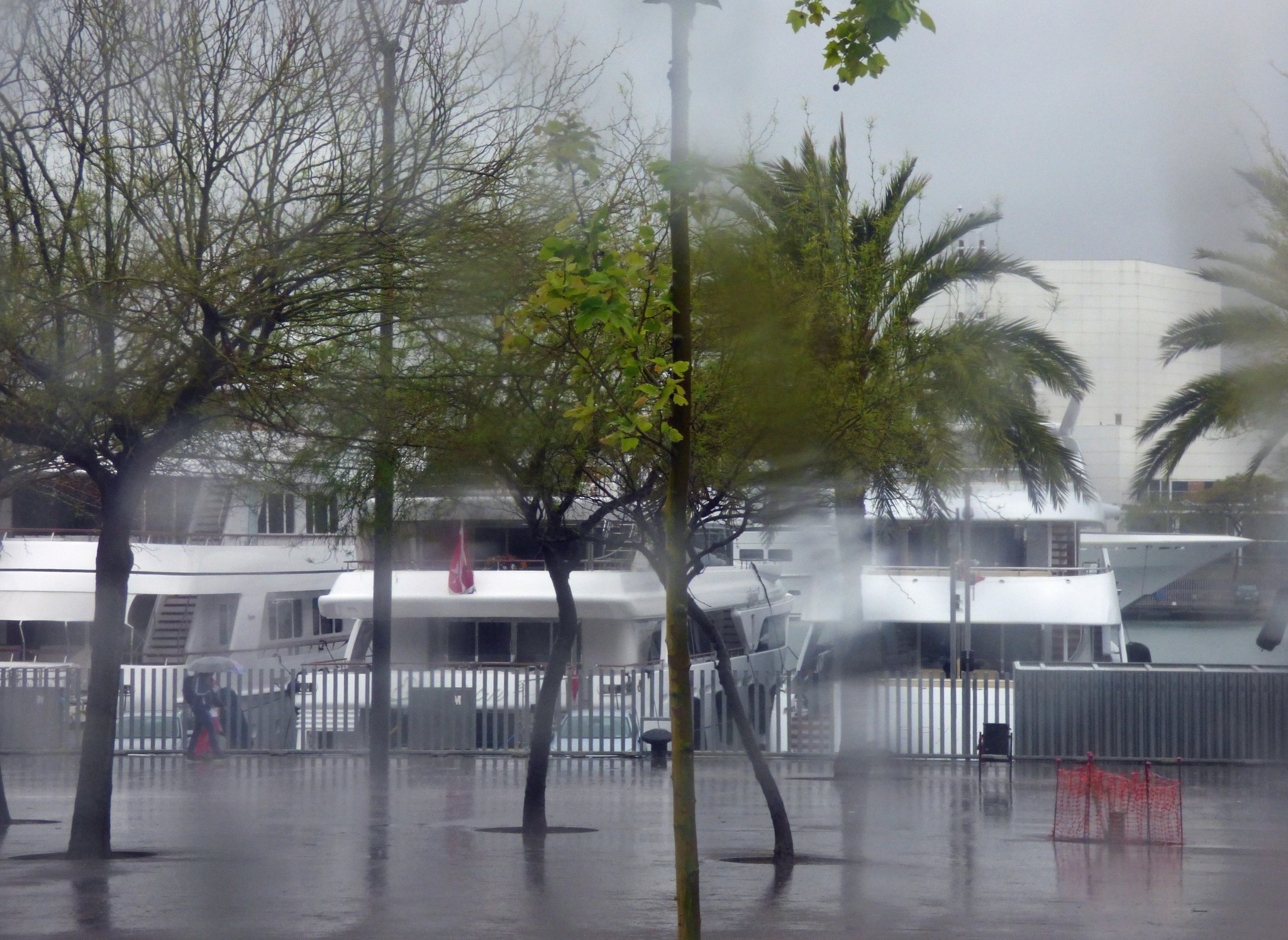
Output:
[187,671,228,761]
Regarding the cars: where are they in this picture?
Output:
[112,710,195,756]
[547,708,649,756]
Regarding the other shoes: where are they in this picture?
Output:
[185,751,203,762]
[212,751,230,760]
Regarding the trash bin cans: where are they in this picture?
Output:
[1,687,70,754]
[411,686,477,752]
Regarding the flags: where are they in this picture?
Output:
[448,520,476,595]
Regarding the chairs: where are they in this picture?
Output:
[978,723,1014,782]
